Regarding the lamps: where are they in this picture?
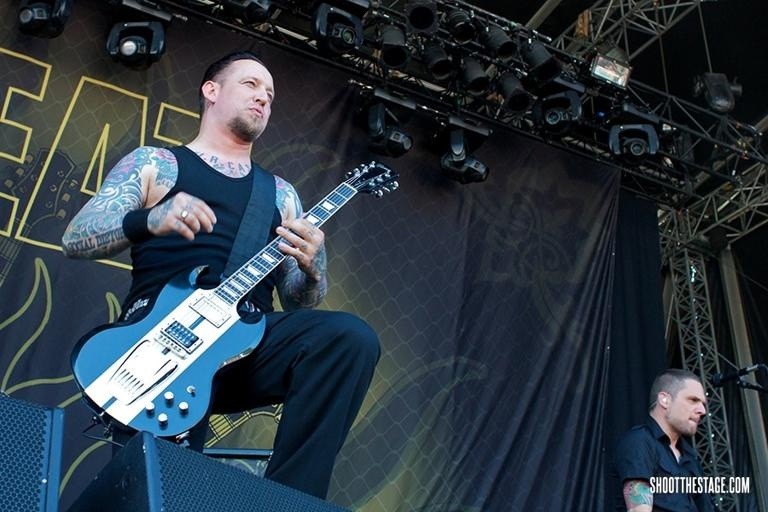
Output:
[11,1,746,194]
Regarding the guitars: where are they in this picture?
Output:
[70,160,399,439]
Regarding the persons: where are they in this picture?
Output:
[615,368,715,512]
[60,50,379,500]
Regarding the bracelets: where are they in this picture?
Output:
[123,205,154,246]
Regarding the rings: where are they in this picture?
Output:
[180,210,189,220]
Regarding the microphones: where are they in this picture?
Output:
[713,365,758,387]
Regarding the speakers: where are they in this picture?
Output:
[0,391,65,511]
[64,431,354,511]
